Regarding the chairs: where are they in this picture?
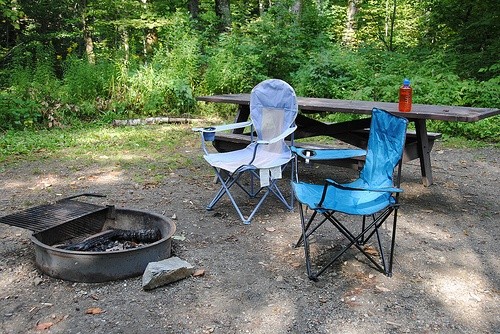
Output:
[291,107,408,280]
[192,78,298,225]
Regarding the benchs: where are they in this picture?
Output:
[211,119,442,185]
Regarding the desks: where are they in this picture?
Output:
[195,94,500,187]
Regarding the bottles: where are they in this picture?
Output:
[399,79,412,112]
